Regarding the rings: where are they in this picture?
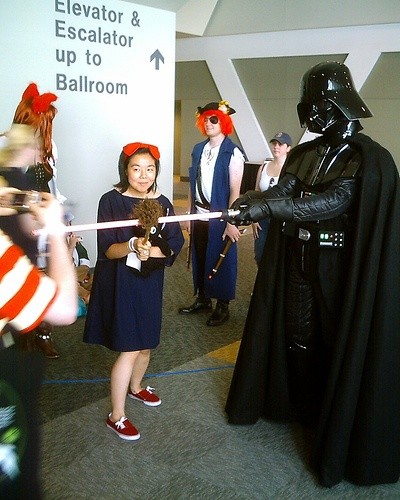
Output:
[142,249,147,254]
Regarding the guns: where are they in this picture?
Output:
[186,231,191,271]
[209,228,247,280]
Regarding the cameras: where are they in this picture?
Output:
[0,189,41,212]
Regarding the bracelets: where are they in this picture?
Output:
[129,237,139,254]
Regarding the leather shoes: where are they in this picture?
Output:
[206,302,230,326]
[179,298,212,315]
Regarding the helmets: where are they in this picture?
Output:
[298,62,374,120]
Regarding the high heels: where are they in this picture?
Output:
[32,329,60,359]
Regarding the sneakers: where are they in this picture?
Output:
[127,386,162,406]
[106,413,141,441]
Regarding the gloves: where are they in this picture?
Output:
[227,187,293,226]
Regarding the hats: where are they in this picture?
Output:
[269,131,292,147]
[197,102,236,116]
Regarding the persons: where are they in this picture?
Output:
[82,142,185,440]
[178,101,245,327]
[251,131,292,268]
[225,62,400,487]
[0,82,80,500]
